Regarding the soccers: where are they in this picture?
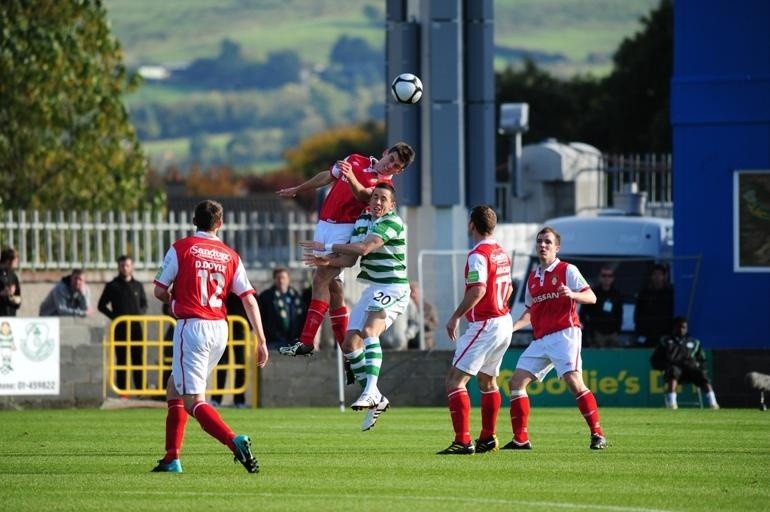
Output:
[393,74,423,104]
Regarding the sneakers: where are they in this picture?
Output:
[149,457,183,473]
[278,340,315,358]
[498,438,533,450]
[343,356,356,386]
[590,433,608,450]
[436,440,474,455]
[474,435,498,453]
[360,394,390,432]
[232,434,260,474]
[351,393,381,411]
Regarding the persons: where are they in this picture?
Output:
[437,203,499,455]
[0,248,22,316]
[207,293,253,408]
[409,278,438,351]
[580,266,624,347]
[257,266,301,351]
[499,226,608,451]
[295,182,410,434]
[650,315,721,409]
[157,302,178,399]
[634,265,674,347]
[272,143,415,385]
[98,255,148,400]
[37,268,92,317]
[148,199,270,474]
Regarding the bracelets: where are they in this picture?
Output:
[323,243,333,251]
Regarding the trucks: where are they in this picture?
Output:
[509,208,678,350]
